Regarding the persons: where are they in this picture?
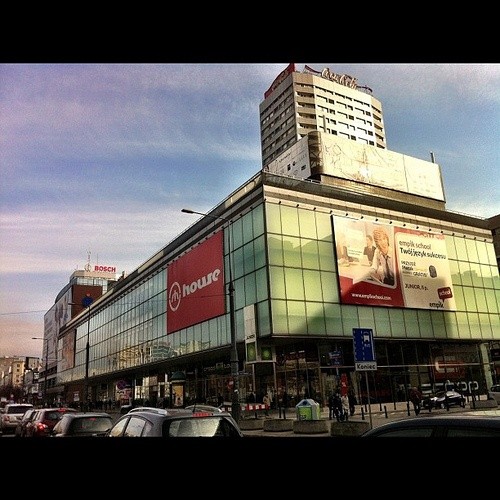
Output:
[218,391,271,416]
[362,226,395,286]
[327,390,357,421]
[50,397,112,411]
[409,385,422,415]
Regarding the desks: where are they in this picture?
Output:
[338,261,383,285]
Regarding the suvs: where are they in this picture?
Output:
[105,404,245,437]
[359,410,500,436]
[0,400,116,438]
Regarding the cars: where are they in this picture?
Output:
[487,384,500,405]
[421,391,468,409]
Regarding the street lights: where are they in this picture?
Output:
[32,337,48,401]
[68,302,90,400]
[182,209,240,404]
